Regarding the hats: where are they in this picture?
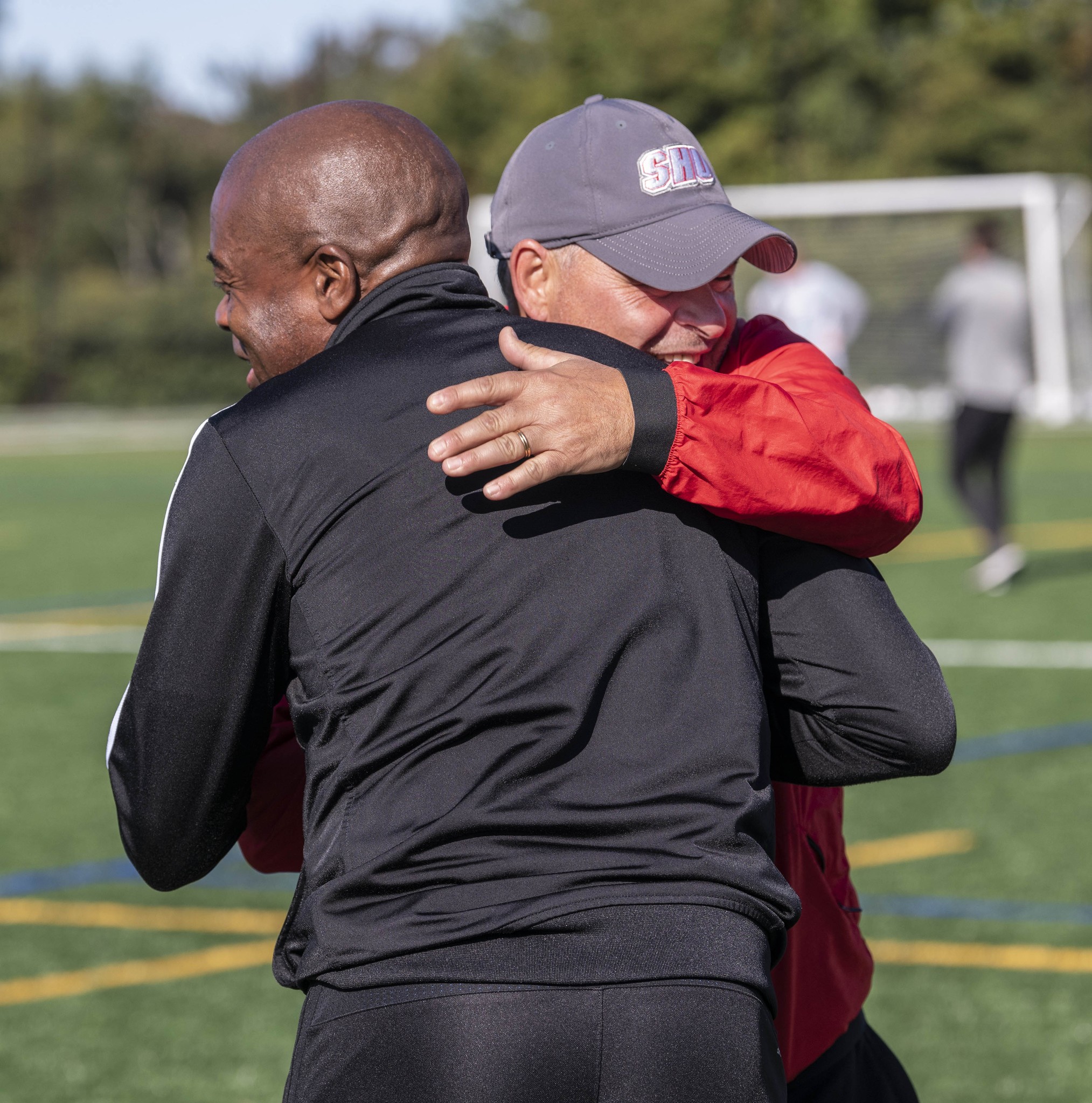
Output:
[483,91,800,297]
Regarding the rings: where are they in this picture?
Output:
[515,429,531,458]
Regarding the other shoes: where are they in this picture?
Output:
[970,541,1028,596]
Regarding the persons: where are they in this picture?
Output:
[236,98,922,1103]
[929,213,1031,596]
[102,99,960,1103]
[748,252,869,377]
